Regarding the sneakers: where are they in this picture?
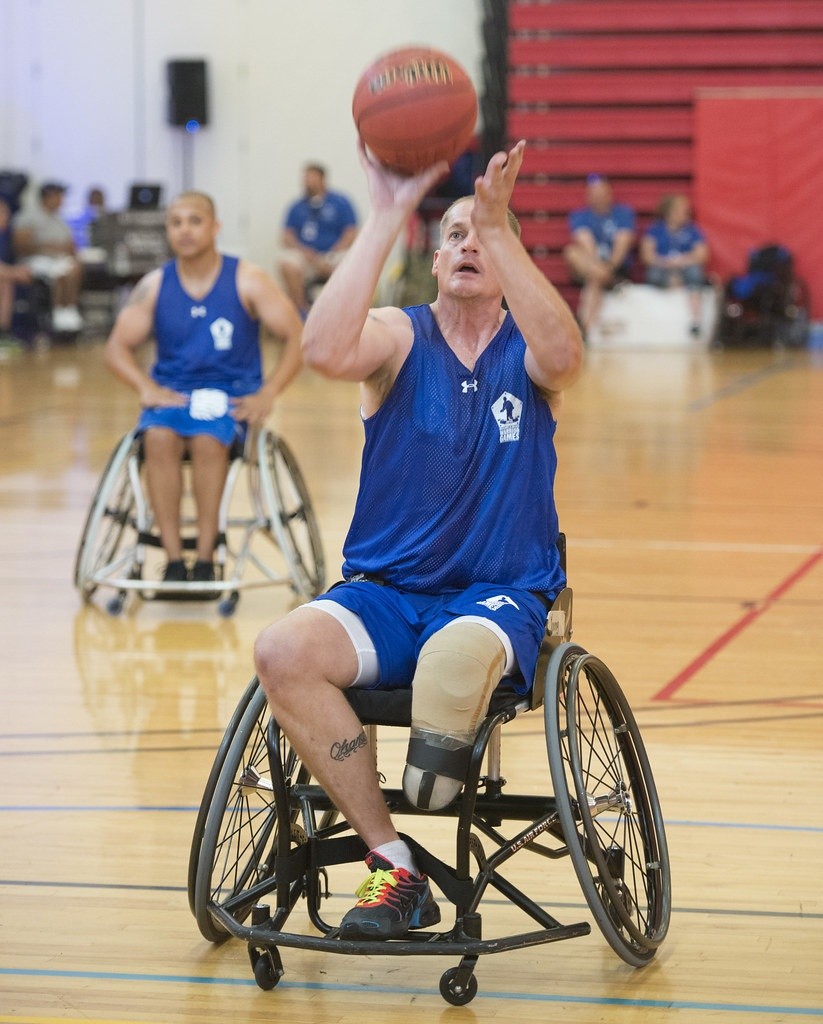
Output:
[338,850,442,942]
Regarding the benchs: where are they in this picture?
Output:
[591,286,718,349]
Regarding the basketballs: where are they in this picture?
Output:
[348,43,482,178]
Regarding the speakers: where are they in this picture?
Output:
[169,61,207,128]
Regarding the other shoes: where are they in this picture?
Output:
[689,325,702,339]
[155,558,186,598]
[188,559,216,597]
[53,302,83,331]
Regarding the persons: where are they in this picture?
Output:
[281,166,358,322]
[253,131,583,942]
[105,190,304,602]
[0,183,105,343]
[564,174,636,334]
[640,193,709,338]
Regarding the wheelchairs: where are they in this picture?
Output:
[74,425,327,619]
[721,244,809,351]
[189,532,670,1007]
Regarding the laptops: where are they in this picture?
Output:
[129,185,159,211]
